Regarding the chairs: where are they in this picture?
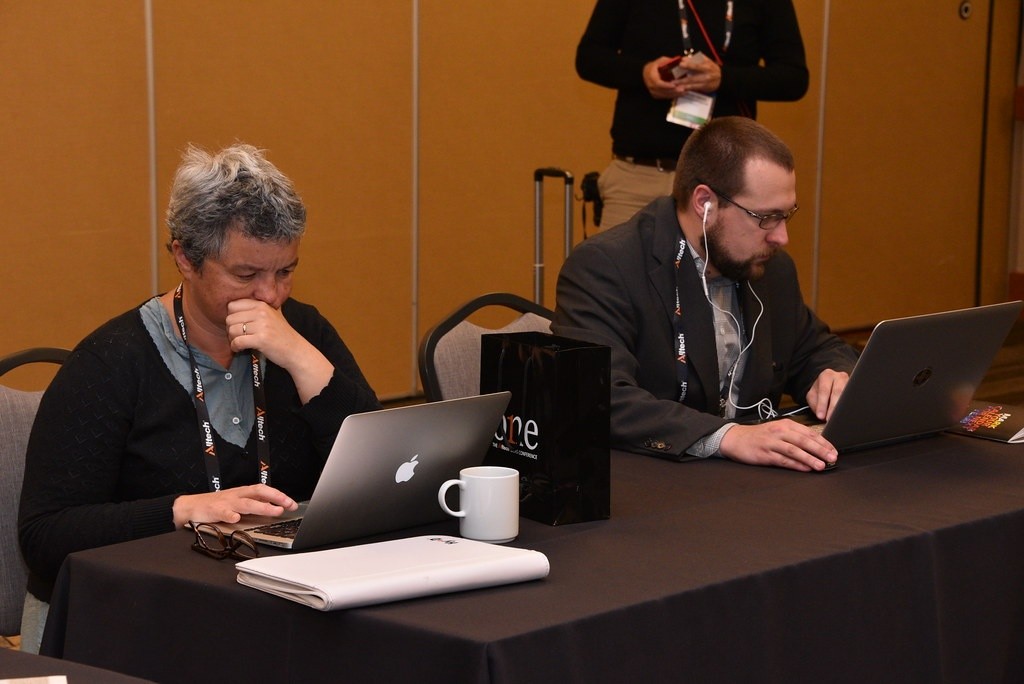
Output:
[0,348,74,643]
[418,289,555,402]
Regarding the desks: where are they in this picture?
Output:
[39,395,1023,683]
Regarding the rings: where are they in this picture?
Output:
[241,322,248,335]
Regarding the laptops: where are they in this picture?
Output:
[760,298,1024,451]
[183,391,511,550]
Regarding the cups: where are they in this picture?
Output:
[438,466,519,544]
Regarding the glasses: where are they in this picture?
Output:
[187,517,261,562]
[694,177,800,231]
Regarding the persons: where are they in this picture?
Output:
[565,0,809,241]
[16,138,383,653]
[551,115,863,475]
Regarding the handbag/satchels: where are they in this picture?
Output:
[478,331,612,528]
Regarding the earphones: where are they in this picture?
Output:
[702,202,712,223]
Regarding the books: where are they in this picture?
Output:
[948,398,1024,447]
[233,532,550,613]
